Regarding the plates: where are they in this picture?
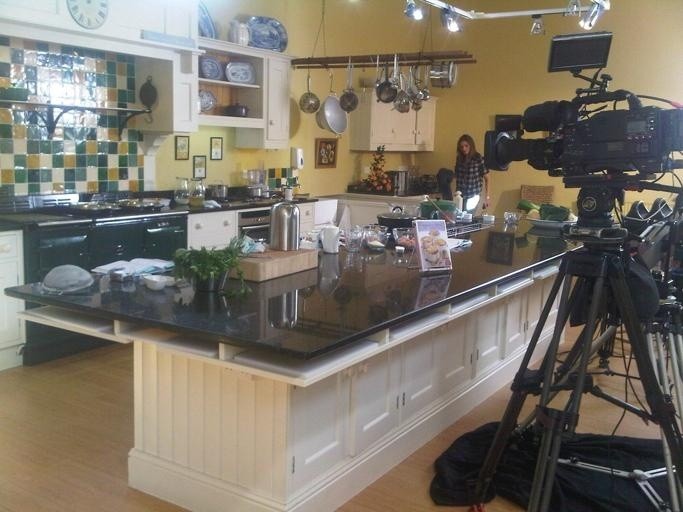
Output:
[199,89,217,111]
[225,61,257,84]
[525,215,577,227]
[198,0,216,39]
[247,16,289,52]
[200,56,223,80]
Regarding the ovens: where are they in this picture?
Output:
[237,209,272,244]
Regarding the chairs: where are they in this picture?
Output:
[520,185,554,214]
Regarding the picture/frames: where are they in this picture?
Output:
[175,135,190,160]
[209,138,224,161]
[315,138,337,168]
[193,156,207,179]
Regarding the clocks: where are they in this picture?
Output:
[66,0,112,29]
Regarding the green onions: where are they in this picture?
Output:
[517,199,578,221]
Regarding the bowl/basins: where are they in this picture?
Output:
[197,269,229,291]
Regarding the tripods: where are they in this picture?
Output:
[475,218,683,511]
[512,269,683,511]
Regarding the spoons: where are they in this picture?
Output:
[338,60,359,112]
[393,72,411,106]
[298,68,320,114]
[41,264,126,292]
[376,60,398,104]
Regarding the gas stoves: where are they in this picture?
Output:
[35,196,167,220]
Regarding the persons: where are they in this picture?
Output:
[449,134,491,216]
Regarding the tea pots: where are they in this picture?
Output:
[226,20,253,46]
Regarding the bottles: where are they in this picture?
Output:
[284,185,293,203]
[188,178,204,209]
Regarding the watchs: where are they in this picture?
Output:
[486,196,490,200]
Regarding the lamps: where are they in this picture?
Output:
[441,0,462,33]
[561,0,580,19]
[402,0,425,21]
[579,0,603,30]
[530,13,547,37]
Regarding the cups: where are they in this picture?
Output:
[364,224,388,251]
[285,188,292,201]
[143,275,168,291]
[189,178,205,207]
[483,215,495,225]
[503,212,518,225]
[343,224,366,253]
[463,214,472,222]
[173,176,189,204]
[392,227,421,268]
[321,226,340,254]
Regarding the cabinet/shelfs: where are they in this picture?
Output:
[349,82,439,151]
[187,191,319,317]
[235,45,294,150]
[0,99,152,141]
[198,35,264,129]
[4,212,588,512]
[0,211,26,373]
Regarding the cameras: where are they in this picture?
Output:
[484,32,683,219]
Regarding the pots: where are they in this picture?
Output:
[315,66,348,135]
[208,185,227,198]
[297,284,316,317]
[390,170,408,196]
[377,205,419,233]
[247,187,263,197]
[333,286,353,321]
[318,254,341,320]
[224,103,249,117]
[368,304,386,324]
